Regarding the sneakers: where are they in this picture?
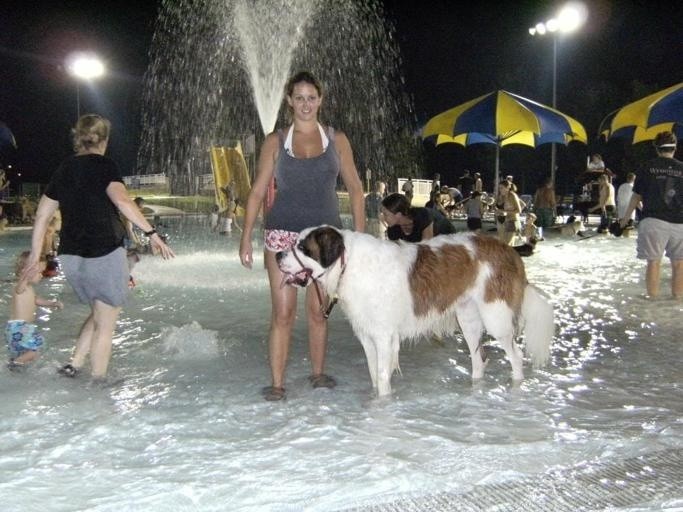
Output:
[8,358,26,372]
[57,364,80,379]
[87,375,125,388]
[307,373,337,389]
[262,386,287,401]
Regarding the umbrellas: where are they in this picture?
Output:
[595,82,683,148]
[420,90,588,206]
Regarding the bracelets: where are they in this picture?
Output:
[143,230,155,236]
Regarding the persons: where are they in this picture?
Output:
[617,131,683,298]
[5,251,63,374]
[400,153,643,248]
[150,215,170,243]
[25,114,175,389]
[124,197,144,258]
[0,121,22,217]
[238,72,365,401]
[40,216,57,259]
[207,205,218,233]
[381,193,456,243]
[364,181,385,237]
[214,200,242,239]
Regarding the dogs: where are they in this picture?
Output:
[275,223,556,398]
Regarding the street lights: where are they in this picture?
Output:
[61,48,105,119]
[528,4,589,185]
